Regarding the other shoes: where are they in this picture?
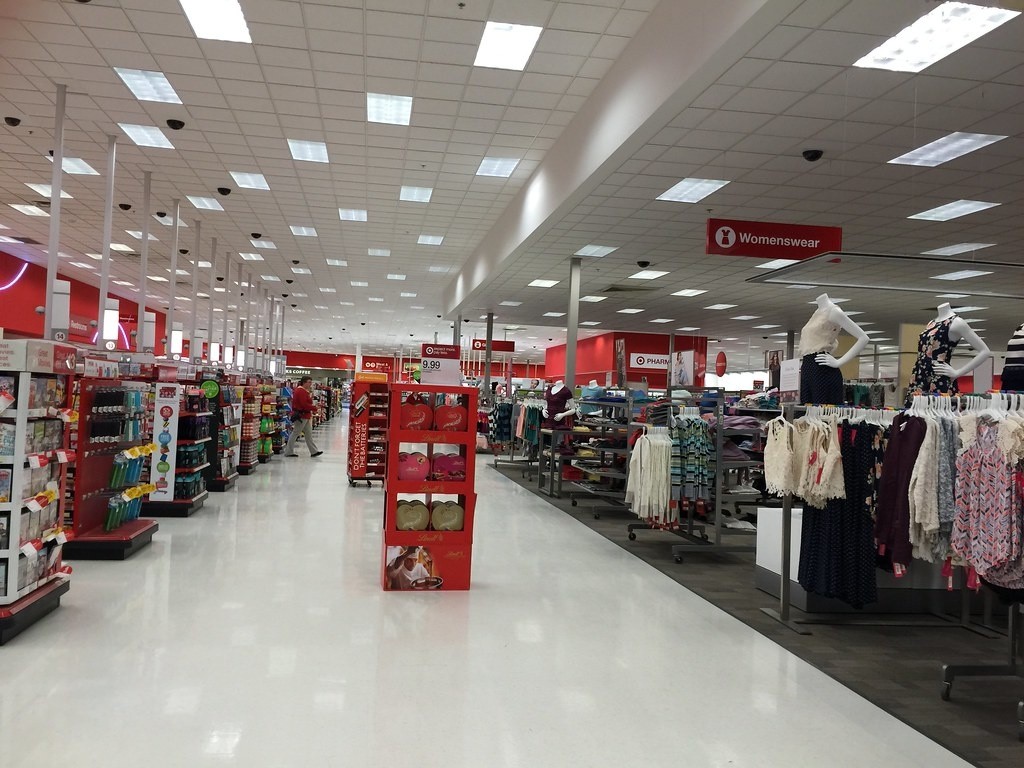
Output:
[311,452,323,457]
[285,454,298,457]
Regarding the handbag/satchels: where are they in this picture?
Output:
[290,413,302,424]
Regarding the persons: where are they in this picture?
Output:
[406,392,424,404]
[541,379,575,429]
[674,352,689,385]
[907,302,993,404]
[576,380,609,420]
[528,379,539,389]
[796,293,870,406]
[999,323,1024,394]
[769,351,781,391]
[284,376,323,458]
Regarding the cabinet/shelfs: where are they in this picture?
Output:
[538,397,632,519]
[151,368,341,507]
[664,386,785,562]
[366,381,474,591]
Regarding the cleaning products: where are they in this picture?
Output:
[257,379,277,456]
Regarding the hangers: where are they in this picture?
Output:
[764,394,1023,442]
[477,396,548,416]
[636,406,708,449]
